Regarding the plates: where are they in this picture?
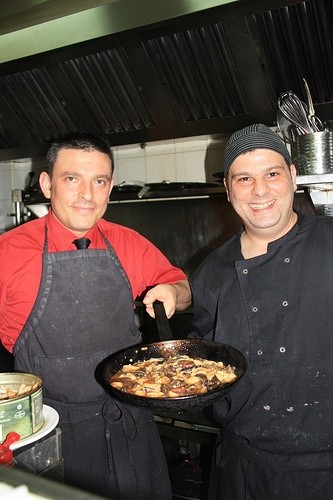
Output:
[0,404,60,451]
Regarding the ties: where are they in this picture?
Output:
[73,237,91,250]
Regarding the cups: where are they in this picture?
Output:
[295,132,333,175]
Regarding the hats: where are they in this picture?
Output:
[223,123,293,177]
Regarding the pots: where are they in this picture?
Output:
[94,300,247,412]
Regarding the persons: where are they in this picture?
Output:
[186,123,333,500]
[0,130,192,500]
